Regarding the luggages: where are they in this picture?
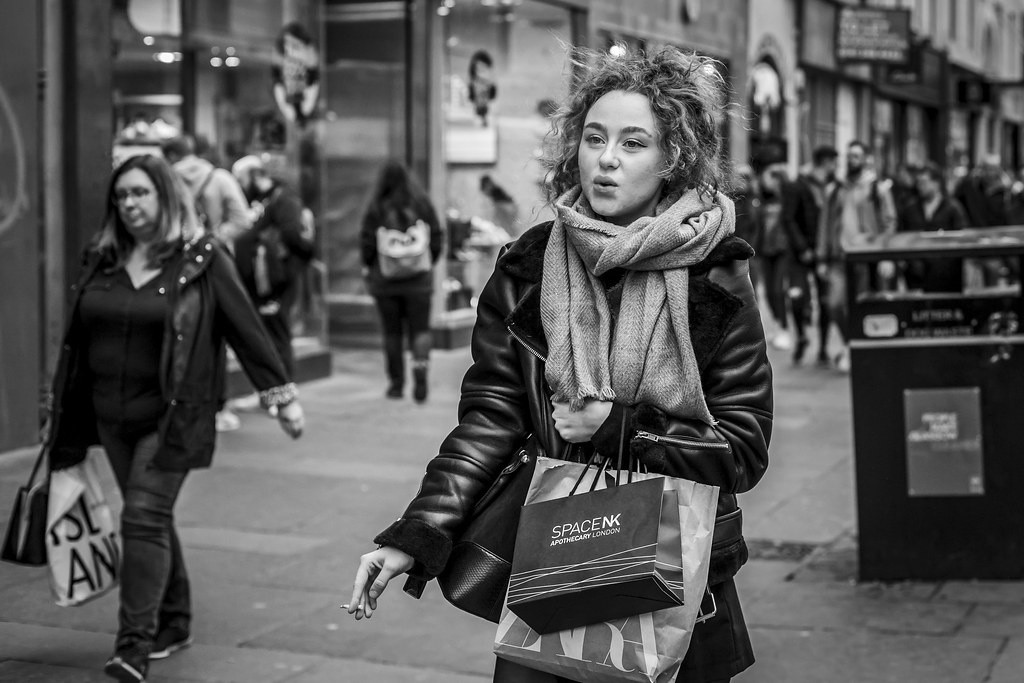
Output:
[415,367,428,401]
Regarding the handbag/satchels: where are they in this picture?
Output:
[235,238,286,314]
[260,206,315,261]
[0,441,49,567]
[45,445,125,607]
[436,406,720,683]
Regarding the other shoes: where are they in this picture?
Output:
[836,349,850,369]
[215,410,240,433]
[794,340,809,360]
[388,385,401,397]
[819,351,827,361]
[773,330,793,349]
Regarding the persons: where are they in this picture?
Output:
[359,160,443,401]
[229,153,314,421]
[444,207,511,306]
[159,137,254,432]
[347,26,774,683]
[720,139,1024,372]
[479,174,519,238]
[38,155,306,683]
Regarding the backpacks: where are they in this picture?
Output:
[374,200,432,278]
[195,164,217,233]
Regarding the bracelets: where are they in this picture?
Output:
[267,395,297,418]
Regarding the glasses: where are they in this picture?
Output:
[848,150,866,161]
[113,186,159,202]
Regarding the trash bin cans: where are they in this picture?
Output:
[840,226,1024,583]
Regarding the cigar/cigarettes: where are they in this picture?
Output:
[340,604,363,609]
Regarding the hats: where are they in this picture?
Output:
[232,154,262,180]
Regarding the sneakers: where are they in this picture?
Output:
[148,632,193,658]
[103,647,147,683]
